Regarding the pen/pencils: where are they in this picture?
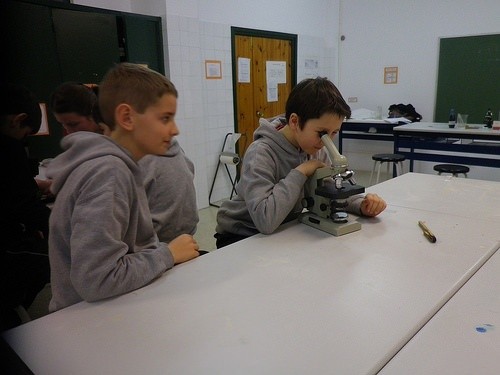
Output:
[417,220,437,243]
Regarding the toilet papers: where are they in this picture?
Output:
[220,155,240,165]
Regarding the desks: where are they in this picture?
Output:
[392,122,500,178]
[338,118,414,172]
[0,172,500,375]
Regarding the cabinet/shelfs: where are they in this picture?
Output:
[0,0,165,161]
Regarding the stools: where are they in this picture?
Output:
[368,154,404,187]
[433,164,470,179]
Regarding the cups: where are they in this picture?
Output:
[457,112,467,130]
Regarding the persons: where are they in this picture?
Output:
[0,63,199,312]
[214,78,382,248]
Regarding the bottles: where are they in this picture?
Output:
[449,109,455,128]
[485,107,493,127]
